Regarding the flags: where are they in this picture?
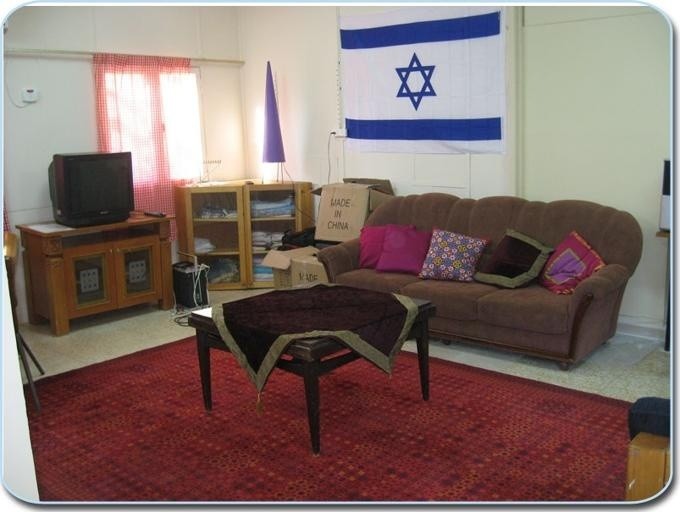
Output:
[339,10,501,143]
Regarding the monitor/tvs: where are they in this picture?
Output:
[48,151,132,228]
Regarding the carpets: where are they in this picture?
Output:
[24,336,635,502]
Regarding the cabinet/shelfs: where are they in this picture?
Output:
[173,178,315,291]
[15,211,175,336]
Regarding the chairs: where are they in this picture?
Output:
[3,231,45,411]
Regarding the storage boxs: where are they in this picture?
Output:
[261,179,395,289]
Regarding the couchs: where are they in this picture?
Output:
[315,192,643,369]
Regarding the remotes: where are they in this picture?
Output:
[144,211,165,218]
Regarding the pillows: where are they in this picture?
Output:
[357,223,605,296]
